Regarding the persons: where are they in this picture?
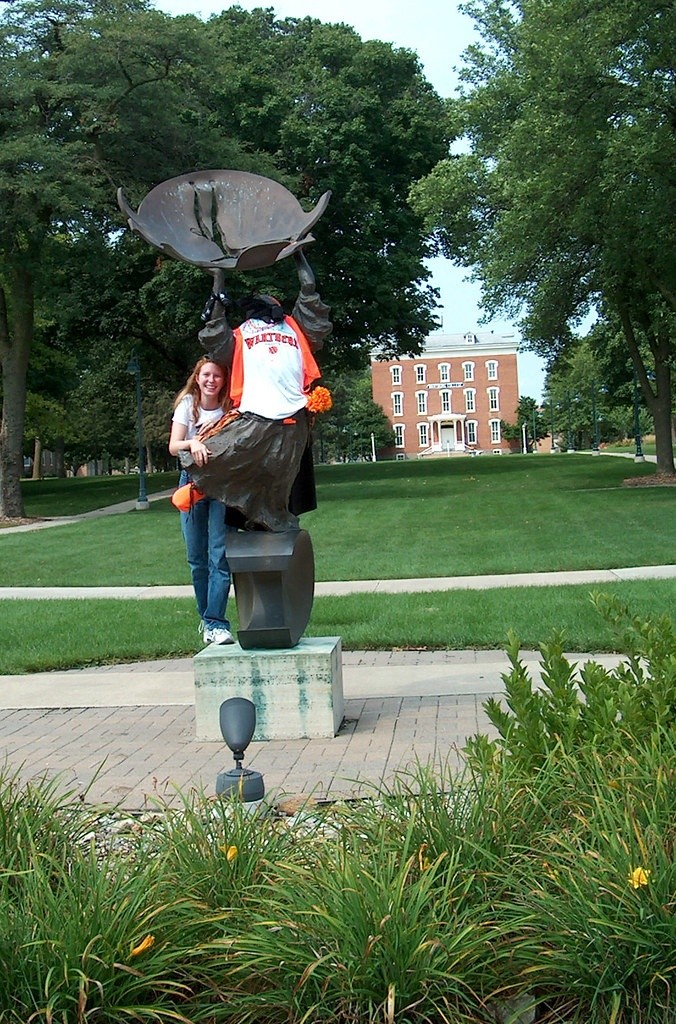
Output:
[172,251,326,532]
[168,358,236,645]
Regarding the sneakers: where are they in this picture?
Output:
[198,620,234,645]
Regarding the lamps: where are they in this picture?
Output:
[215,696,266,803]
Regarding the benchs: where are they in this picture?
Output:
[464,451,477,457]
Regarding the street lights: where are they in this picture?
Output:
[590,378,609,457]
[342,427,352,463]
[531,405,543,454]
[125,348,150,510]
[550,399,562,454]
[567,390,581,455]
[632,365,654,464]
[354,429,363,462]
